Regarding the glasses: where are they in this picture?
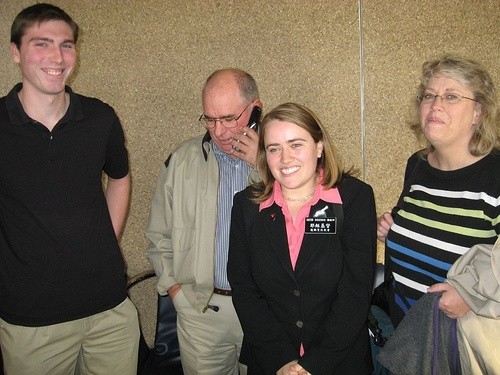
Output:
[198,100,254,129]
[415,93,482,105]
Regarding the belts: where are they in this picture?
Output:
[212,288,232,297]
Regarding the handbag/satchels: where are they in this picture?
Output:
[366,280,403,374]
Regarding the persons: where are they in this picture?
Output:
[144,67,270,375]
[377,56,499,375]
[0,0,140,375]
[227,102,377,375]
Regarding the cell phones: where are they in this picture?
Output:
[233,105,263,153]
